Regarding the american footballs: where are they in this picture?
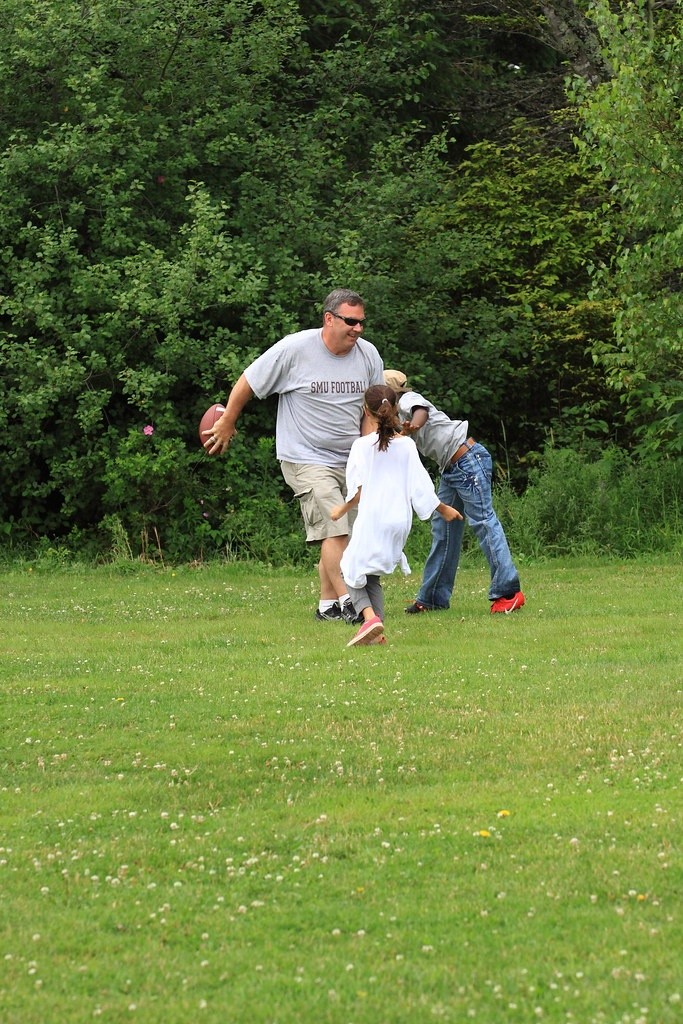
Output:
[199,403,234,455]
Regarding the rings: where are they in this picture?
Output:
[210,436,215,442]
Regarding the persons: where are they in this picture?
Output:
[330,385,464,647]
[383,370,524,614]
[201,289,384,625]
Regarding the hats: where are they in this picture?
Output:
[383,370,412,392]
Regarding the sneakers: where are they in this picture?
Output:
[490,591,525,614]
[404,602,429,614]
[315,602,343,623]
[347,614,384,647]
[370,634,386,644]
[340,597,365,625]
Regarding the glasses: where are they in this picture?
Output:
[326,310,366,326]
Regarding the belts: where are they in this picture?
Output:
[451,438,475,464]
